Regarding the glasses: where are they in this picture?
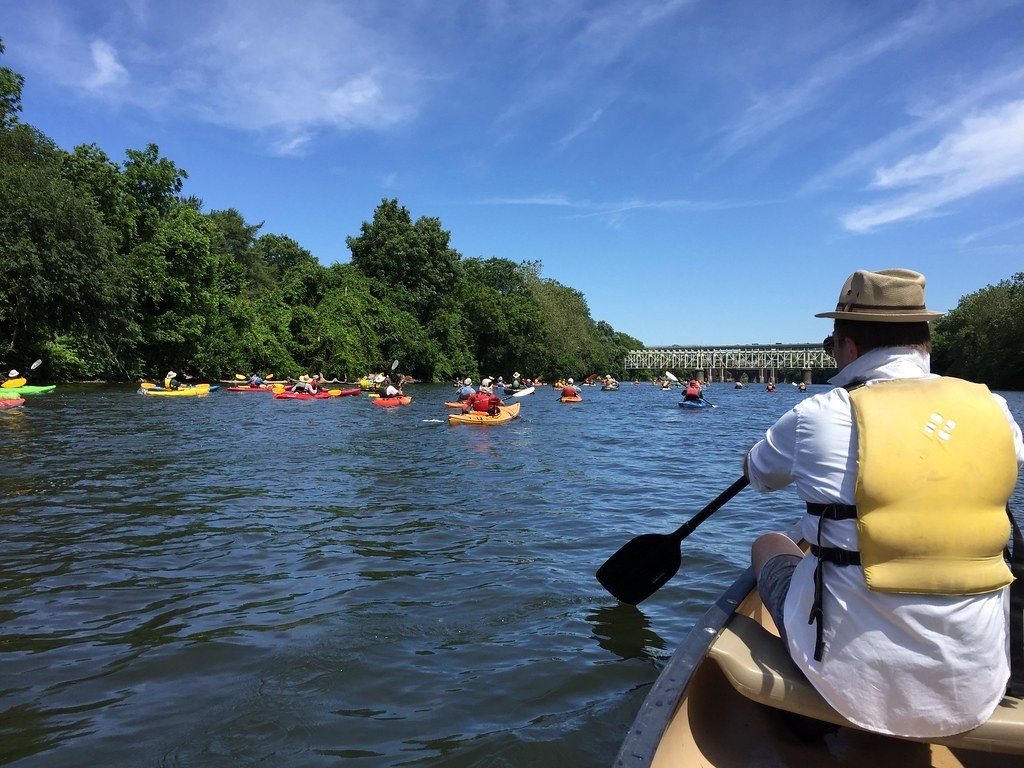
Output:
[823,334,855,358]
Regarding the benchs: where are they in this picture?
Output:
[706,612,1024,756]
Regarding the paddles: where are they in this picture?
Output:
[17,359,42,378]
[665,370,721,409]
[792,382,809,393]
[596,475,750,605]
[501,386,535,401]
[140,383,210,389]
[235,373,284,389]
[441,371,463,387]
[385,359,399,377]
[272,388,342,396]
[556,374,598,402]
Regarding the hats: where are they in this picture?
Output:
[8,369,19,377]
[299,374,313,382]
[167,371,177,378]
[375,375,386,382]
[568,378,573,383]
[480,379,493,391]
[691,379,695,384]
[815,270,947,323]
[313,375,319,379]
[464,378,471,385]
[513,372,520,378]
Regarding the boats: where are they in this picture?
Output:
[767,389,775,392]
[209,386,220,392]
[583,383,596,386]
[445,401,473,408]
[493,384,512,388]
[662,387,671,391]
[555,388,582,403]
[601,383,618,391]
[448,402,520,425]
[679,401,706,408]
[141,383,210,396]
[0,385,56,396]
[372,396,412,407]
[238,384,266,389]
[614,513,1023,768]
[227,384,294,392]
[635,382,640,385]
[274,388,361,400]
[0,393,25,410]
[504,388,521,395]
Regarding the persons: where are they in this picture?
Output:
[744,267,1023,739]
[512,372,539,389]
[662,381,669,388]
[246,373,267,388]
[682,380,704,402]
[162,371,187,391]
[456,378,475,402]
[560,378,581,398]
[360,374,386,390]
[8,370,22,381]
[497,380,505,387]
[379,378,402,398]
[766,383,776,390]
[601,374,618,385]
[291,375,330,395]
[798,382,806,391]
[462,379,505,416]
